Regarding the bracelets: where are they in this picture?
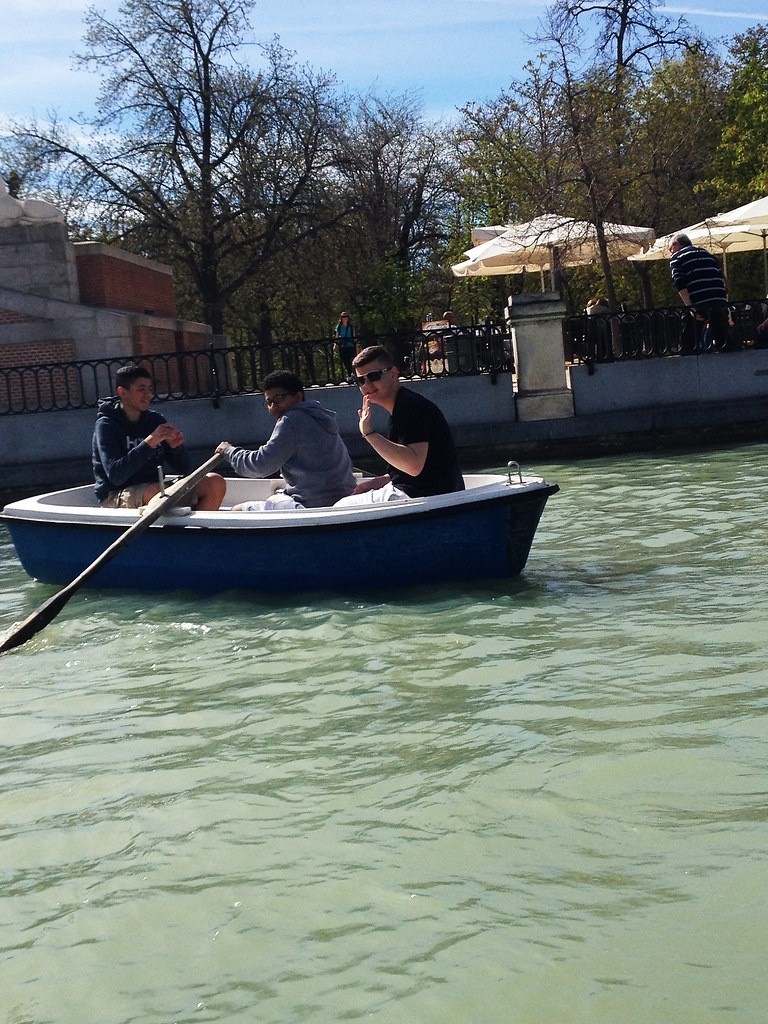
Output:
[364,431,376,439]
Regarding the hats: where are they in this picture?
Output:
[340,312,348,317]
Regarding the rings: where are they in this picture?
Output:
[179,432,184,435]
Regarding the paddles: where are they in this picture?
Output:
[1,453,225,655]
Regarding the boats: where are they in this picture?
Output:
[0,461,560,591]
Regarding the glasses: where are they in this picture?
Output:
[263,392,292,408]
[352,366,393,388]
[342,316,348,318]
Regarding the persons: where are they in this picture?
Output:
[585,297,612,338]
[331,345,465,507]
[331,311,360,383]
[91,366,227,511]
[474,311,515,372]
[440,310,465,338]
[666,235,733,352]
[215,370,358,512]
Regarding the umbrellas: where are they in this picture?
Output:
[448,214,655,294]
[628,195,768,300]
